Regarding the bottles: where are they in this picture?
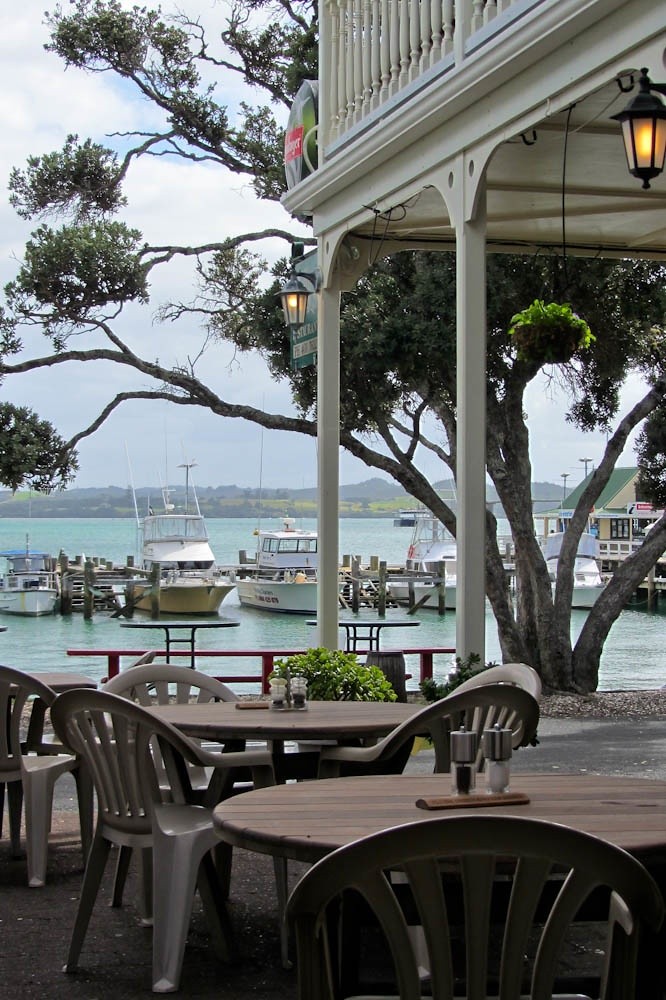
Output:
[484,722,513,795]
[290,676,308,708]
[268,678,286,708]
[450,726,477,796]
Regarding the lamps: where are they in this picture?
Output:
[608,68,666,189]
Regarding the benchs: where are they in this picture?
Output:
[67,646,457,694]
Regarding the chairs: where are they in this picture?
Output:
[1,650,666,1000]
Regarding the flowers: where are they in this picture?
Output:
[508,299,596,363]
[274,262,320,332]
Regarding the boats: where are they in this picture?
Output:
[389,512,457,610]
[541,534,604,609]
[0,486,58,617]
[123,438,236,613]
[235,394,318,615]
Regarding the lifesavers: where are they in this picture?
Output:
[408,545,414,559]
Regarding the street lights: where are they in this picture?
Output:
[561,474,570,501]
[580,457,593,479]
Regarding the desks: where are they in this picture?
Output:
[139,699,465,974]
[119,621,241,689]
[305,619,420,654]
[6,670,99,859]
[212,773,666,1000]
